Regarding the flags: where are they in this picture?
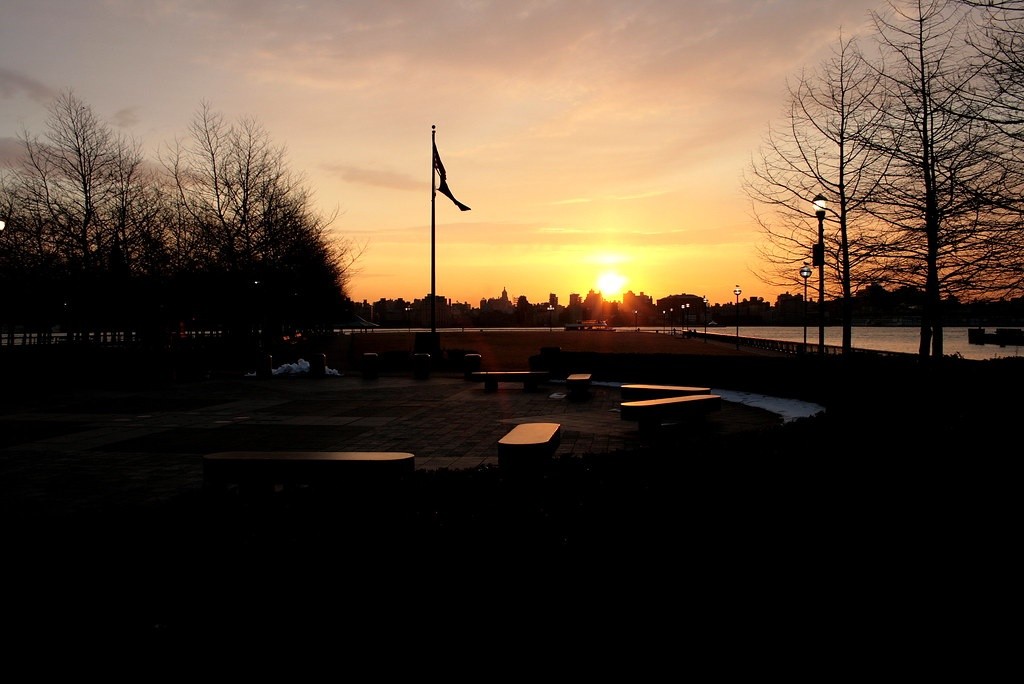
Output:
[433,143,470,211]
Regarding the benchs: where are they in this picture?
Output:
[620,382,709,400]
[566,373,592,396]
[619,394,726,435]
[203,451,417,483]
[470,371,552,392]
[495,423,562,468]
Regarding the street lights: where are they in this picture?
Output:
[663,310,666,335]
[546,305,554,333]
[799,261,813,354]
[811,194,828,356]
[686,302,690,330]
[733,284,743,350]
[634,310,637,332]
[681,303,685,331]
[405,305,411,332]
[669,307,673,335]
[702,295,709,344]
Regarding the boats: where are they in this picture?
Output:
[564,320,617,331]
